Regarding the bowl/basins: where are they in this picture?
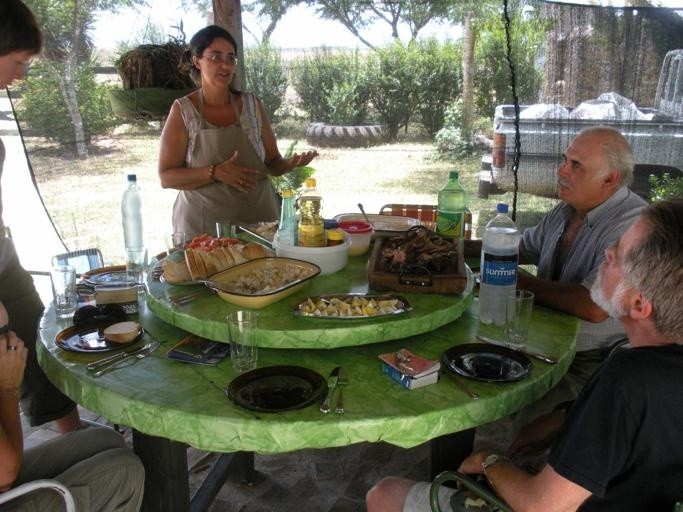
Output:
[206,256,322,308]
[268,228,352,278]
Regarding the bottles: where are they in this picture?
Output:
[120,173,143,250]
[277,177,345,247]
[436,170,466,244]
[48,264,77,320]
[480,204,519,326]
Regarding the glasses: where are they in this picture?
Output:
[198,55,238,65]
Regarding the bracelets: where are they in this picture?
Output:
[209,164,218,183]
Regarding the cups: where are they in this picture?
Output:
[165,230,185,255]
[124,245,148,293]
[338,220,372,256]
[212,218,236,237]
[502,287,534,343]
[225,308,259,373]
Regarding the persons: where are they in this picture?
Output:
[158,25,319,247]
[366,197,683,512]
[464,127,651,454]
[0,0,87,436]
[0,330,145,512]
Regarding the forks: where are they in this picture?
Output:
[332,366,350,415]
[90,341,165,379]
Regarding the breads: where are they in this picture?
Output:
[163,242,266,282]
[104,320,140,343]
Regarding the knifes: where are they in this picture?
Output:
[442,367,480,403]
[320,363,341,414]
[477,332,560,367]
[167,285,216,306]
[83,340,161,370]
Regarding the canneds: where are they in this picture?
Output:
[327,229,343,246]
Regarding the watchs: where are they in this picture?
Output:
[481,454,510,480]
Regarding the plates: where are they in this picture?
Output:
[441,344,535,384]
[293,292,414,321]
[159,259,204,287]
[225,365,327,412]
[54,318,145,352]
[331,213,420,233]
[81,265,130,287]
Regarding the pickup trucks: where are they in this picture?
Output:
[478,46,683,205]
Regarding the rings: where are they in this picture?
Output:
[7,346,17,350]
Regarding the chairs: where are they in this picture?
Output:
[428,466,515,512]
[1,480,76,511]
[378,202,472,240]
